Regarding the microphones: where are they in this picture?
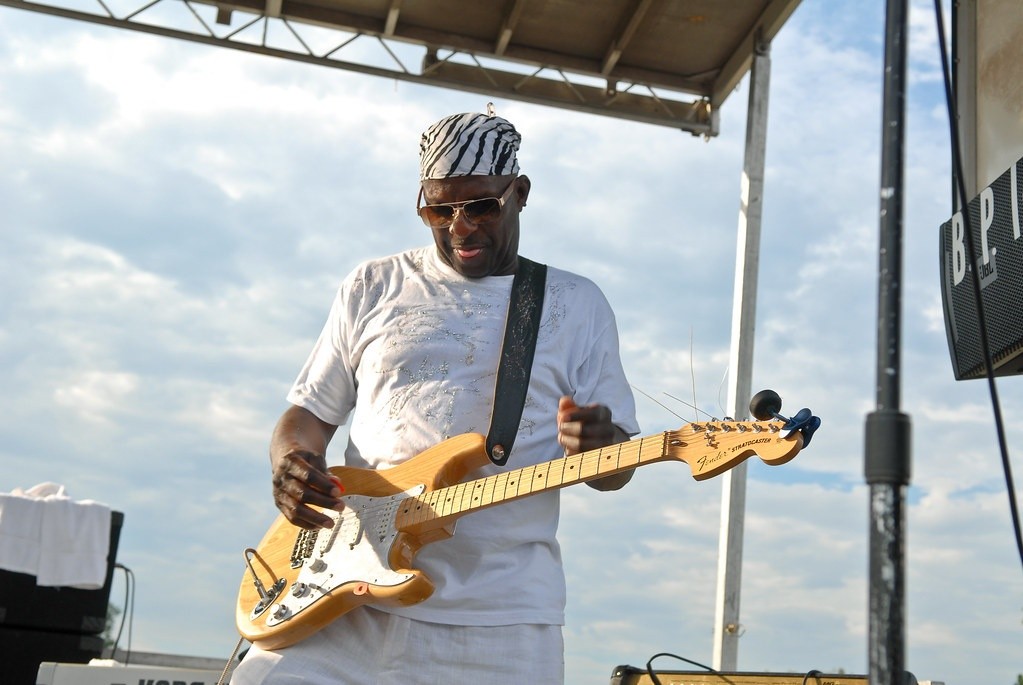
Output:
[749,390,791,424]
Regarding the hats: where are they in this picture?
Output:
[419,114,522,182]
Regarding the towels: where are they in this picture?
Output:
[0,481,112,591]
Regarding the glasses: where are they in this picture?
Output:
[417,176,521,228]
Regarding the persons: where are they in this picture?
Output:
[229,112,640,685]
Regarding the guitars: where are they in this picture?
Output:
[232,389,821,653]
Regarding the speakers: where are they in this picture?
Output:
[1,511,124,634]
[939,157,1023,380]
